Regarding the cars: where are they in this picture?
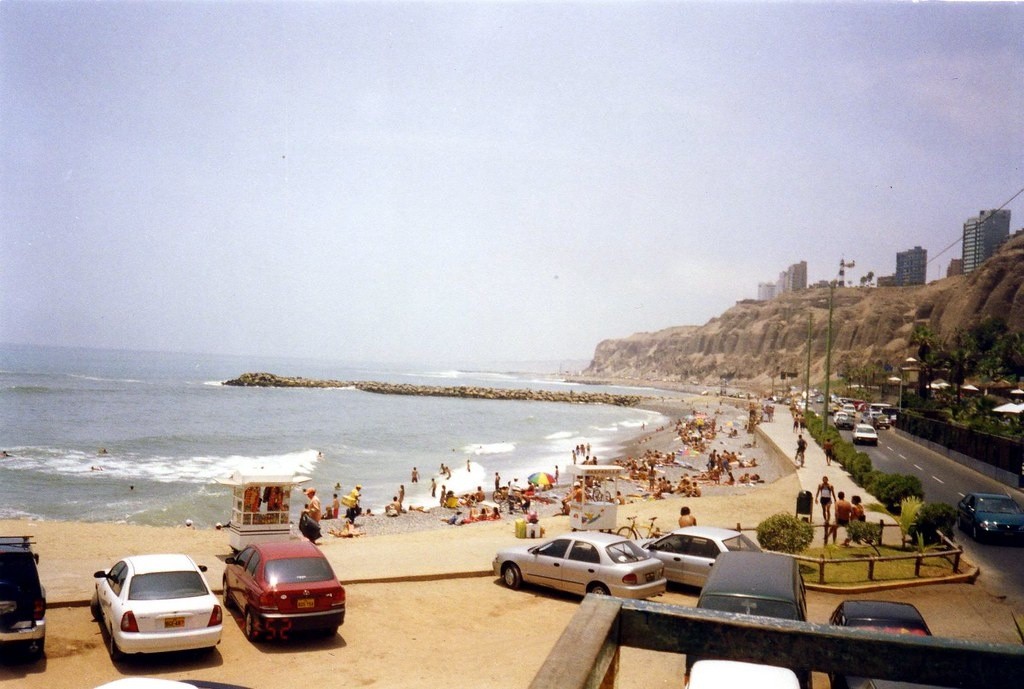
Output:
[851,423,878,446]
[697,550,806,623]
[91,554,223,668]
[958,493,1024,546]
[632,526,764,588]
[828,599,934,638]
[773,387,897,430]
[492,531,666,600]
[222,540,345,644]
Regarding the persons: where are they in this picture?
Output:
[302,481,375,545]
[766,405,775,422]
[815,476,864,547]
[430,459,542,525]
[91,466,104,471]
[3,451,12,457]
[789,403,806,434]
[98,447,107,454]
[411,467,419,483]
[317,451,322,458]
[679,507,697,547]
[824,438,834,465]
[216,523,221,529]
[385,485,404,515]
[542,465,559,491]
[129,485,134,492]
[185,518,192,529]
[553,417,760,517]
[795,434,807,467]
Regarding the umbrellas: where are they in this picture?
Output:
[528,472,555,486]
[679,448,700,455]
[725,420,741,426]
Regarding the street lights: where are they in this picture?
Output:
[823,278,836,432]
[805,309,814,414]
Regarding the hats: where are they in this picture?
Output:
[574,482,581,487]
[356,484,362,488]
[304,487,316,495]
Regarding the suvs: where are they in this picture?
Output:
[0,536,47,666]
